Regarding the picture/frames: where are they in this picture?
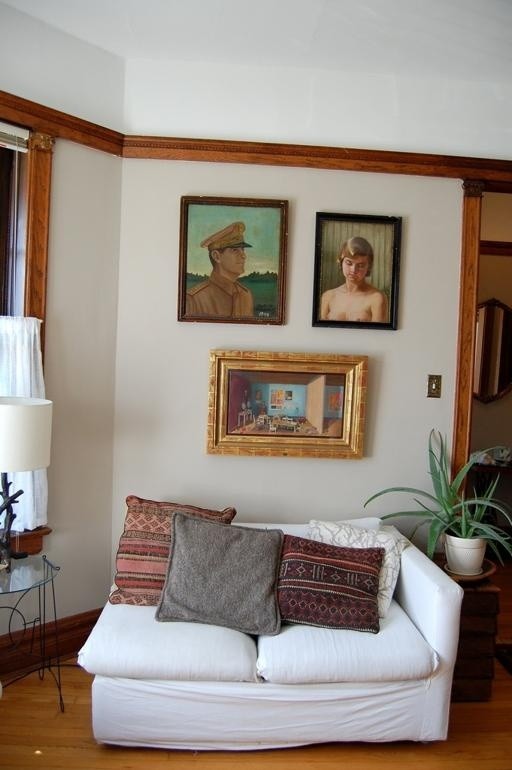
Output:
[206,350,368,461]
[177,196,288,326]
[312,211,402,331]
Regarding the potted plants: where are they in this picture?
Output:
[364,431,512,579]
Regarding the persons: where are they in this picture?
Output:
[322,237,386,321]
[185,221,250,321]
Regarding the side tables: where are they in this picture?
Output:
[0,556,65,713]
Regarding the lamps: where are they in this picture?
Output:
[0,397,53,571]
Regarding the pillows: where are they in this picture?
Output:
[109,497,236,606]
[305,520,411,617]
[278,533,382,635]
[154,512,283,636]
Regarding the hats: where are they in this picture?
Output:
[201,222,253,251]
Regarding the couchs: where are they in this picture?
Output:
[79,521,463,752]
